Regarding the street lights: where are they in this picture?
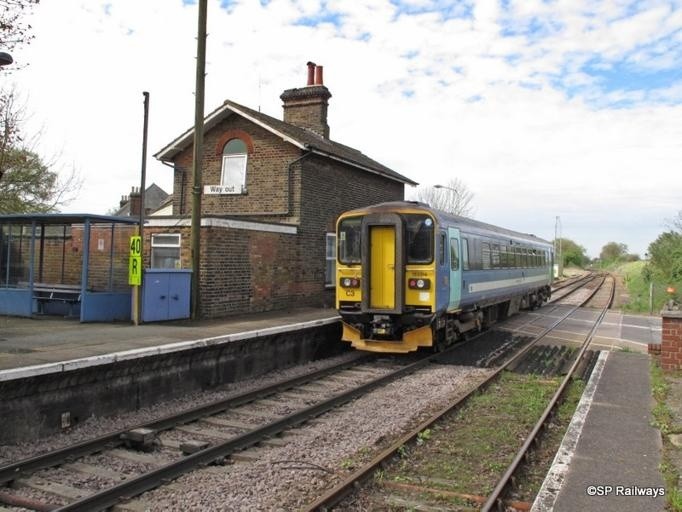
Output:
[434,185,460,214]
[0,51,12,68]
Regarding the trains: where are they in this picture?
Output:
[329,205,557,357]
